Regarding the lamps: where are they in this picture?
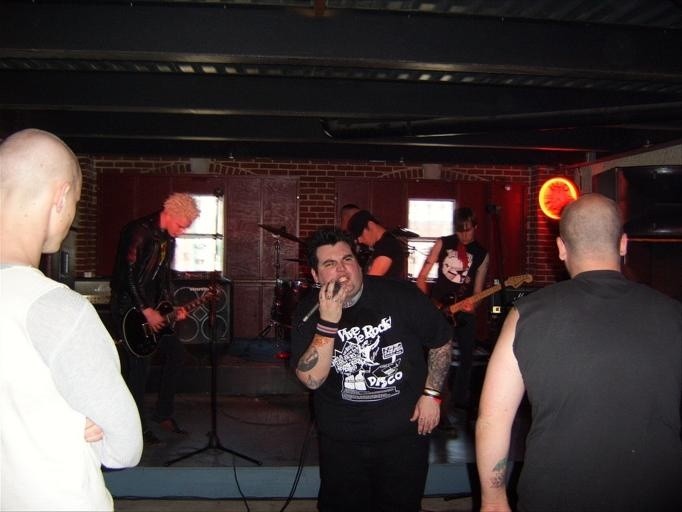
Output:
[539,176,579,223]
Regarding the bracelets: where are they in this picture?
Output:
[314,320,339,339]
[422,388,444,399]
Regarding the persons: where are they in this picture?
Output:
[335,204,369,258]
[474,194,682,512]
[416,207,488,431]
[110,193,200,442]
[285,226,455,512]
[1,128,144,512]
[348,210,403,276]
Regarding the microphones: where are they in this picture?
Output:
[213,274,236,289]
[296,282,340,329]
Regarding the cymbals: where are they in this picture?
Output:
[257,221,305,247]
[383,227,420,239]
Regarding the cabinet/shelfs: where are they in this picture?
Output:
[333,175,407,280]
[591,165,682,300]
[96,173,301,340]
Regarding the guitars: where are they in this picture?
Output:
[429,274,533,328]
[122,289,225,358]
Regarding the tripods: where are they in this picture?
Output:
[164,293,271,466]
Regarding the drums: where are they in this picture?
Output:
[271,278,322,330]
